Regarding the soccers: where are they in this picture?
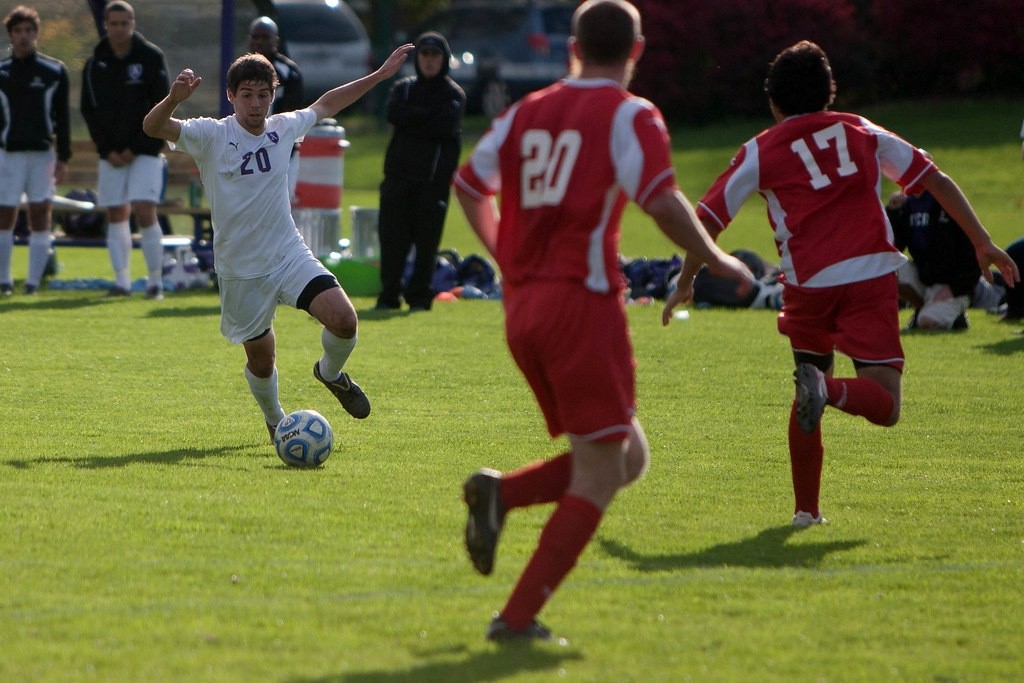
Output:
[274,410,334,469]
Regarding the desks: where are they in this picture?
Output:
[157,207,214,243]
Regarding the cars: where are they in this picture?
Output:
[377,0,576,121]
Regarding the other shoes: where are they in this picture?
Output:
[0,283,13,294]
[23,283,37,297]
[104,286,131,297]
[143,285,162,301]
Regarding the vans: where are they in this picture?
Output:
[273,0,374,112]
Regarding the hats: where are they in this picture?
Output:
[416,37,444,52]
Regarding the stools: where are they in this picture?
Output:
[159,238,209,288]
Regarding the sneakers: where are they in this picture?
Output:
[313,362,371,420]
[484,618,561,650]
[265,420,278,445]
[462,470,501,576]
[789,364,829,437]
[795,508,829,528]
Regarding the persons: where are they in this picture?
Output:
[0,6,71,293]
[372,30,465,311]
[248,16,304,205]
[143,44,415,445]
[662,41,1020,529]
[884,148,982,330]
[80,0,172,300]
[453,0,757,641]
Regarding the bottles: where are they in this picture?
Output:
[190,166,203,208]
